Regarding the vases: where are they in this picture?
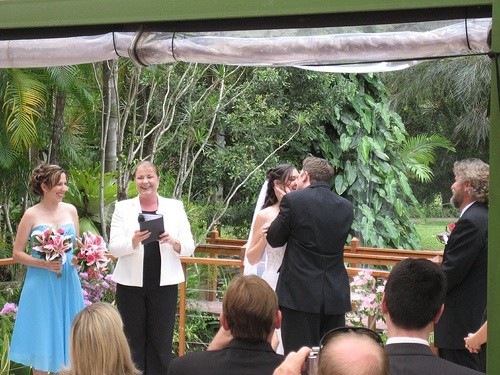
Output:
[6,366,32,375]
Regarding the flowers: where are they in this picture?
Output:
[31,224,73,278]
[346,272,387,334]
[1,303,30,368]
[75,272,116,310]
[72,230,112,274]
[448,223,455,231]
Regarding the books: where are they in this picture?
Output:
[139,213,164,244]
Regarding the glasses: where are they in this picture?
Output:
[320,326,383,348]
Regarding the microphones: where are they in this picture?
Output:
[138,215,145,225]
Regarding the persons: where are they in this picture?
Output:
[382,258,483,375]
[433,157,489,372]
[9,165,86,375]
[272,328,388,375]
[243,164,300,355]
[266,157,354,375]
[58,302,144,375]
[464,306,487,373]
[167,274,286,375]
[109,162,195,375]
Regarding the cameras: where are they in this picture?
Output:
[307,345,324,375]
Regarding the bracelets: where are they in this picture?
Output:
[173,240,179,247]
